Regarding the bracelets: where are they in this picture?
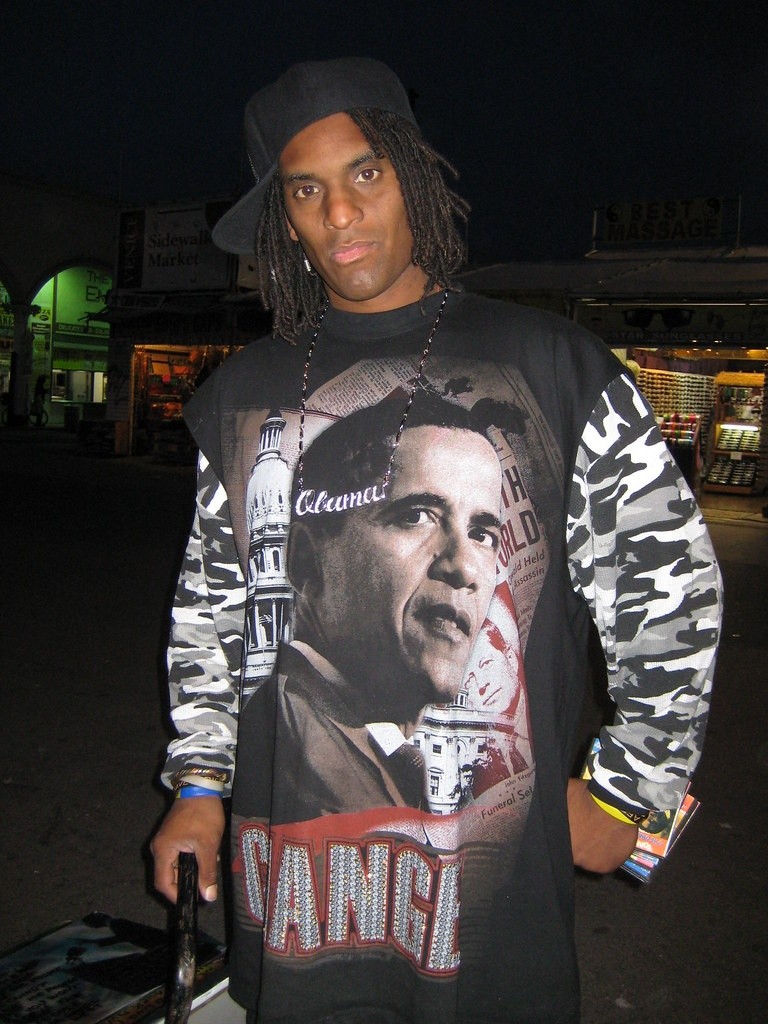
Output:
[173,782,225,799]
[579,762,653,827]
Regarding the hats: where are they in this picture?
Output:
[210,57,419,254]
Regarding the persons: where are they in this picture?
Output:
[225,392,506,843]
[142,54,729,1024]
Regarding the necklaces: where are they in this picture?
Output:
[293,291,453,517]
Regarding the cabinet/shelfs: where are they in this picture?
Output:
[140,390,183,450]
[80,419,129,458]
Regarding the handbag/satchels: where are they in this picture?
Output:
[30,402,41,416]
